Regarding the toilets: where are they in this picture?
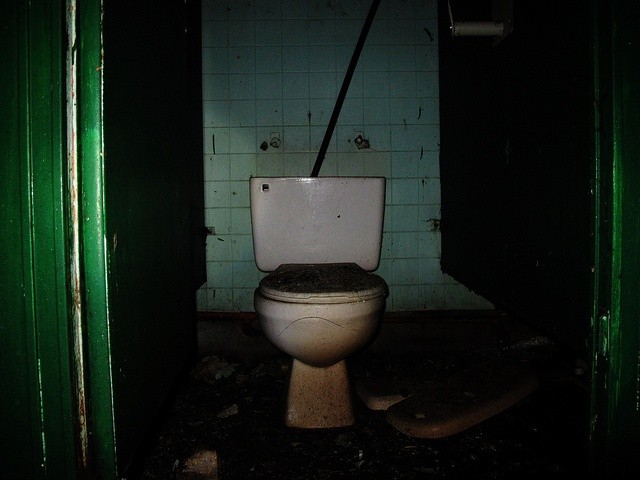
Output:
[237,169,396,433]
